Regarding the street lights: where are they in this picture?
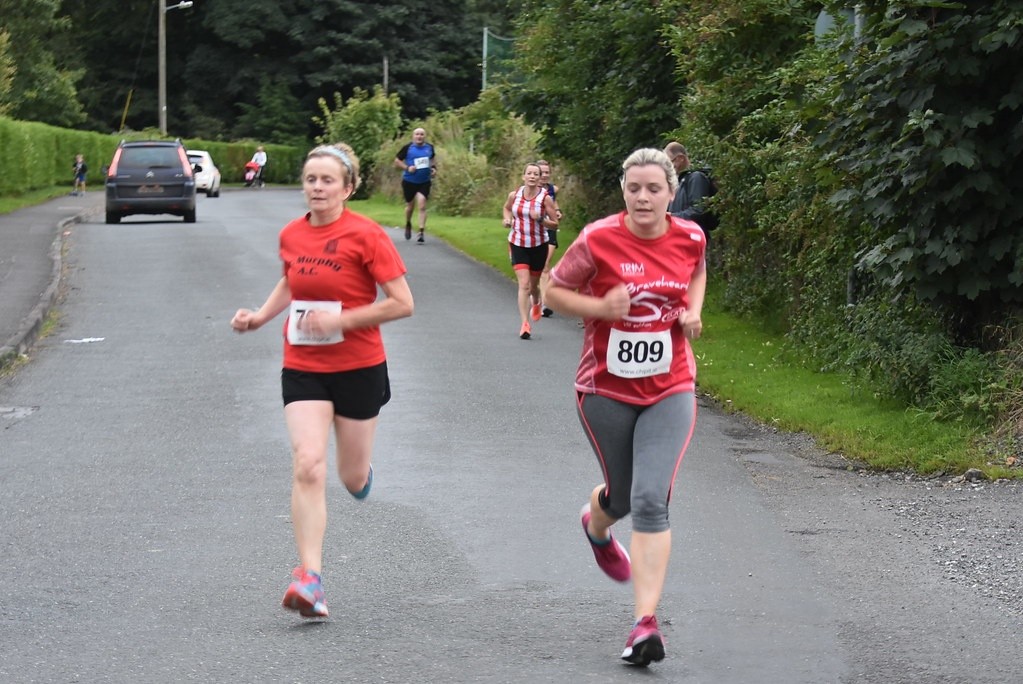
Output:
[158,1,194,136]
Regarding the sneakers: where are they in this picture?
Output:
[541,304,553,317]
[581,503,632,581]
[405,225,411,239]
[531,295,540,322]
[622,617,665,666]
[282,568,328,618]
[347,465,373,500]
[418,232,424,242]
[520,324,531,339]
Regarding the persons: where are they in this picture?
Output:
[662,142,708,233]
[502,163,560,339]
[393,128,437,242]
[535,160,563,317]
[230,143,415,618]
[250,146,268,188]
[72,154,88,196]
[544,147,706,666]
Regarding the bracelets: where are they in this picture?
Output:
[537,216,543,223]
[406,165,410,171]
[556,209,562,214]
[431,165,436,168]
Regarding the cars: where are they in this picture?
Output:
[187,149,221,198]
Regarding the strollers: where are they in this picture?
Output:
[244,162,260,187]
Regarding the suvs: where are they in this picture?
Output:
[101,138,203,225]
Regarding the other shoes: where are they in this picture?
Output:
[70,192,78,196]
